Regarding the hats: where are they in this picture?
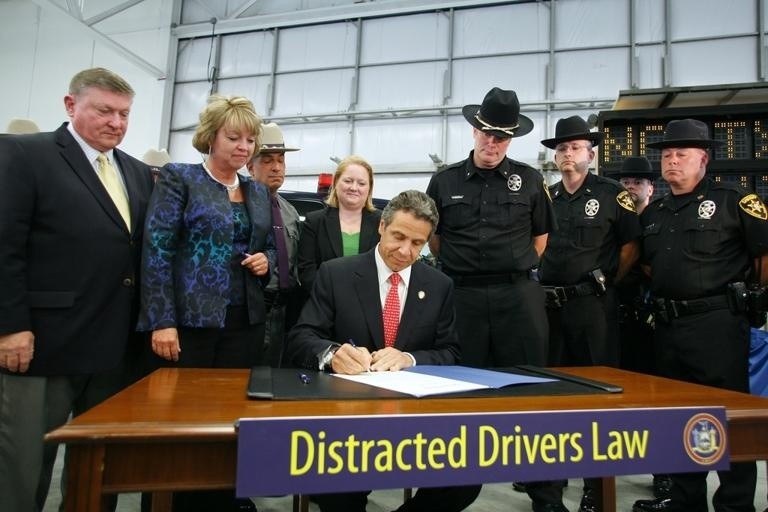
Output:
[541,116,604,150]
[605,156,661,182]
[646,119,725,150]
[462,87,534,137]
[253,124,300,156]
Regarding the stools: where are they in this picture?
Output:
[290,487,415,512]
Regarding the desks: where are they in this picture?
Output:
[42,364,763,511]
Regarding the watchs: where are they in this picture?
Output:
[323,346,340,369]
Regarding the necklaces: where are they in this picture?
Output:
[200,165,244,191]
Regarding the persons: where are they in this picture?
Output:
[632,147,767,510]
[539,138,643,511]
[416,126,571,511]
[612,176,673,496]
[244,121,302,365]
[296,156,383,295]
[0,68,153,512]
[137,91,276,511]
[284,189,482,512]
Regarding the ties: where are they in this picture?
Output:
[384,274,402,346]
[272,197,290,288]
[95,152,130,231]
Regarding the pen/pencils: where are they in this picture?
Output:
[349,339,374,372]
[298,371,311,384]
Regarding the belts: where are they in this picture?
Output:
[548,285,594,300]
[454,271,531,286]
[668,297,729,318]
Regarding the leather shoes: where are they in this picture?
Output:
[577,495,601,512]
[632,496,671,512]
[531,501,569,512]
